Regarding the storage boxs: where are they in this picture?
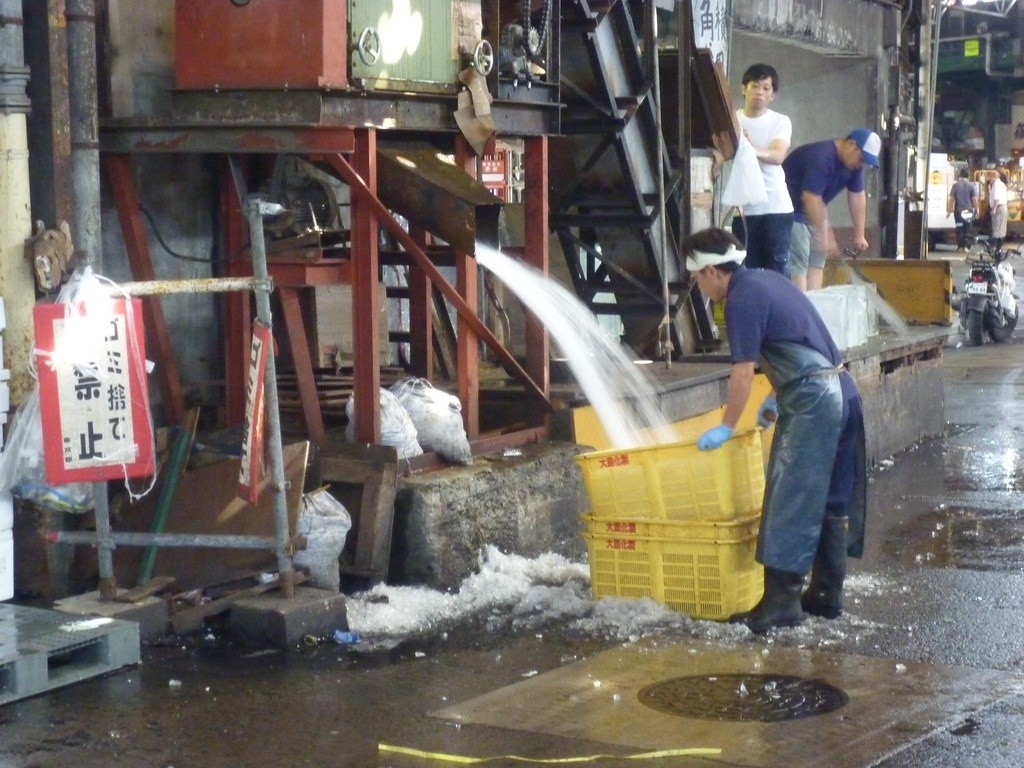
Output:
[574,423,770,621]
[979,197,1022,221]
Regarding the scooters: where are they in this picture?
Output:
[957,224,1024,345]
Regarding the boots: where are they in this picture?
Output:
[729,565,806,631]
[996,238,1003,251]
[990,238,998,247]
[801,516,849,618]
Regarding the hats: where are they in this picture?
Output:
[851,129,882,168]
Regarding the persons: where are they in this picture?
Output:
[782,127,882,291]
[735,64,794,277]
[947,168,979,253]
[986,171,1008,250]
[987,160,1007,193]
[683,227,867,634]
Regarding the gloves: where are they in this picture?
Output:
[756,397,778,427]
[697,424,733,450]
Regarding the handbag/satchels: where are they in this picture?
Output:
[997,286,1016,318]
[720,127,768,206]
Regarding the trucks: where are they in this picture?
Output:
[927,152,970,252]
